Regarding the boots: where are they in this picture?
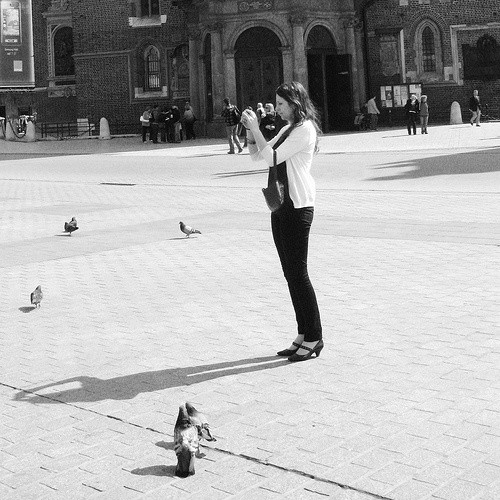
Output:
[425,127,428,134]
[421,128,424,134]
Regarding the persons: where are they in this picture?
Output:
[240,81,325,362]
[242,102,277,147]
[220,97,244,155]
[406,95,419,135]
[468,89,482,127]
[368,94,380,132]
[141,101,197,144]
[418,95,430,134]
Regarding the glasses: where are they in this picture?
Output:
[265,107,269,109]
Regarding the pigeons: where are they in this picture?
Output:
[178,220,202,239]
[64,222,79,236]
[184,402,218,443]
[30,285,44,308]
[172,405,199,479]
[68,216,78,227]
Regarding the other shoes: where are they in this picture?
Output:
[476,125,480,127]
[471,122,473,125]
[238,148,243,153]
[227,151,235,154]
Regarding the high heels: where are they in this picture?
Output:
[276,342,302,356]
[288,339,324,361]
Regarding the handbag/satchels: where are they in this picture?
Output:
[140,116,150,122]
[262,179,285,212]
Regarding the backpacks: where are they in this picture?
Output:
[232,109,241,125]
